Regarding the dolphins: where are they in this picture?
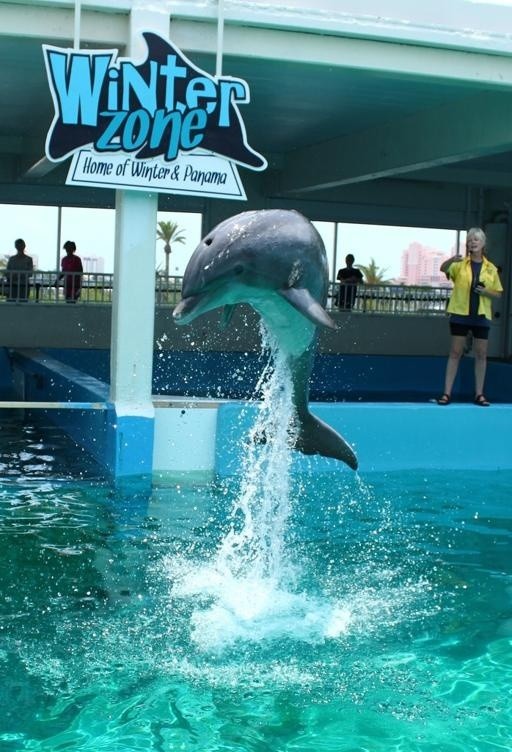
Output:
[172,208,358,471]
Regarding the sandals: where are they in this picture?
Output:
[437,394,450,405]
[473,394,490,406]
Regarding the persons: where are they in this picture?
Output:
[438,227,505,407]
[54,240,83,303]
[335,254,364,312]
[5,238,33,302]
